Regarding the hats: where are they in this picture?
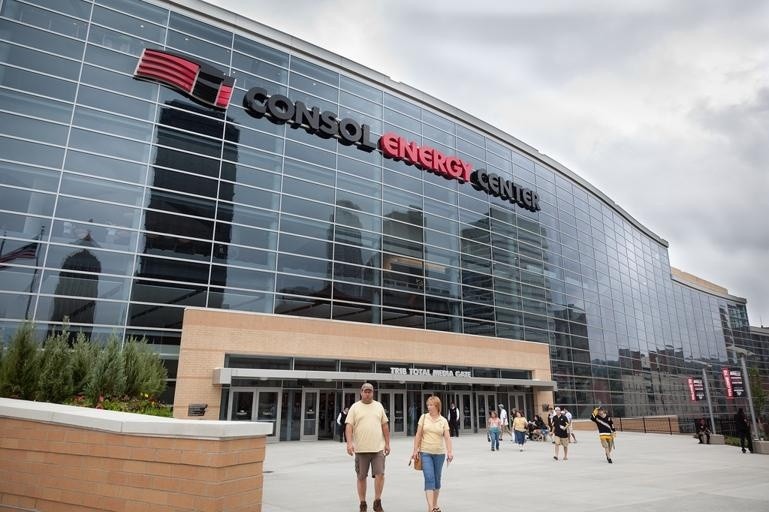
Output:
[362,383,373,391]
[498,404,504,408]
[555,407,560,411]
[597,406,603,410]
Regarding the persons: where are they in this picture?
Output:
[697,407,769,453]
[407,401,419,436]
[499,404,513,441]
[412,395,454,512]
[510,404,579,461]
[344,382,391,512]
[337,406,349,442]
[488,410,502,451]
[590,406,617,464]
[448,401,460,438]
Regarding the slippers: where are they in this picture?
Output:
[433,508,441,512]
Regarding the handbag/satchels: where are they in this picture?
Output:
[488,435,490,442]
[414,454,422,470]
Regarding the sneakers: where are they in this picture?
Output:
[373,498,384,512]
[742,449,746,453]
[554,456,558,460]
[360,501,367,512]
[606,453,613,463]
